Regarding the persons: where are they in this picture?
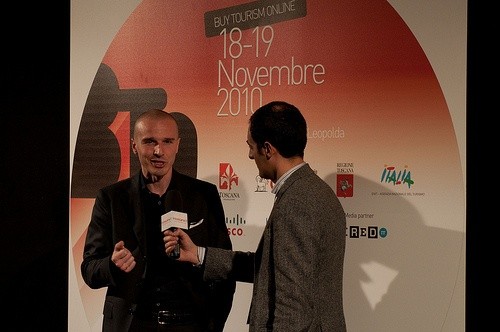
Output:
[162,101,348,332]
[80,108,238,332]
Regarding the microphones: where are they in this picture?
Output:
[161,189,188,259]
